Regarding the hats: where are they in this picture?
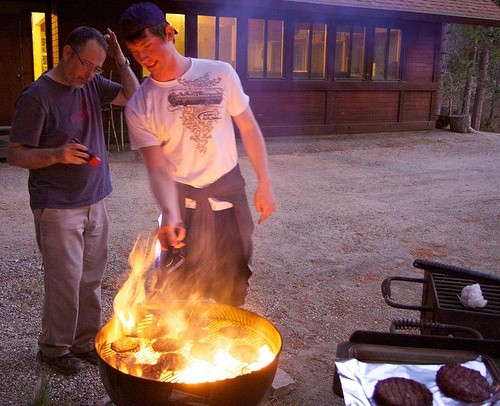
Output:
[119,3,178,39]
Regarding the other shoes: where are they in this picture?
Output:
[72,348,100,365]
[37,349,85,374]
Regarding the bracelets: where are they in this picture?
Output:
[116,56,130,68]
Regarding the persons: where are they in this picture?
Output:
[123,0,277,309]
[6,25,142,378]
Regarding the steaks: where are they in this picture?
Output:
[435,364,491,402]
[111,316,260,381]
[373,377,434,406]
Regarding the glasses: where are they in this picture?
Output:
[71,48,105,76]
